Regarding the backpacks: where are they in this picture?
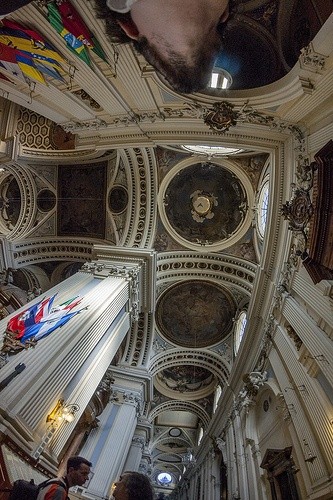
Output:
[7,477,66,500]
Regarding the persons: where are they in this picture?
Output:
[112,471,154,500]
[37,456,92,500]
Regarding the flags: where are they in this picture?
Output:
[6,290,91,345]
